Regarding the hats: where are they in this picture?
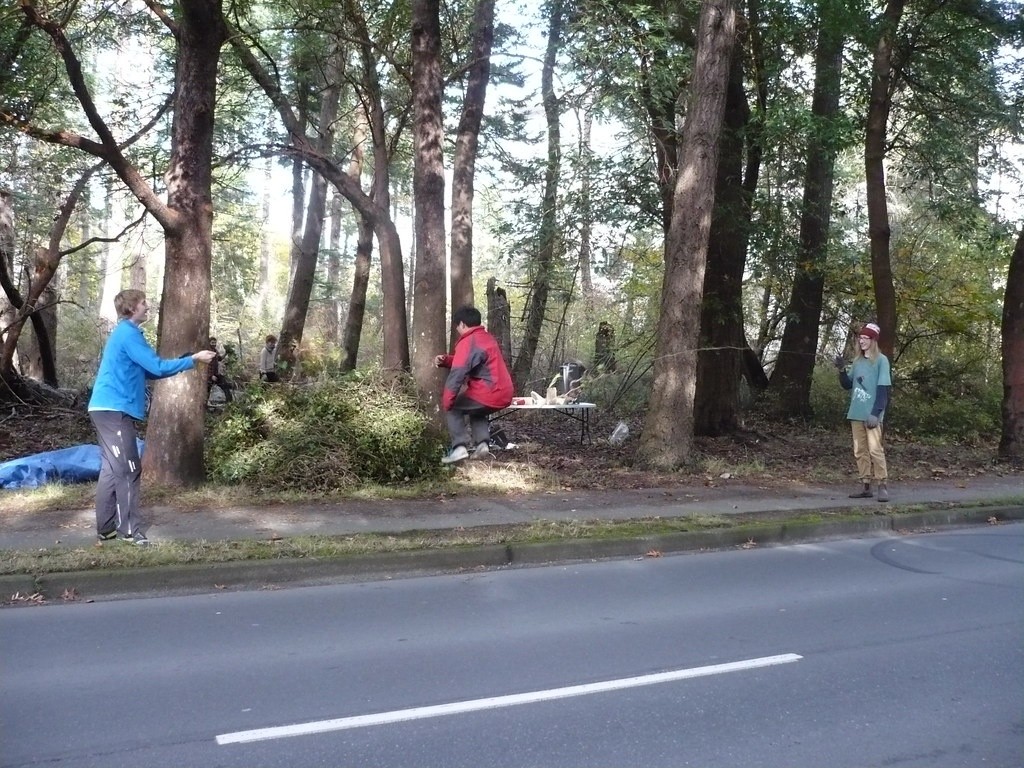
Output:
[855,323,880,341]
[266,335,276,342]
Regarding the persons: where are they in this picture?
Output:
[834,323,892,503]
[87,289,217,548]
[260,335,278,382]
[434,305,514,463]
[205,337,232,408]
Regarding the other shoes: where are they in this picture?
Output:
[97,529,117,541]
[470,442,489,460]
[878,483,889,502]
[116,531,150,546]
[848,484,873,497]
[441,447,468,463]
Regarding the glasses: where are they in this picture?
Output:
[857,337,871,342]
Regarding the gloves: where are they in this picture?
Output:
[866,415,878,428]
[833,356,844,372]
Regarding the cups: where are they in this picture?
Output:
[547,387,557,404]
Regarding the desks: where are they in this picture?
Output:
[489,397,596,445]
